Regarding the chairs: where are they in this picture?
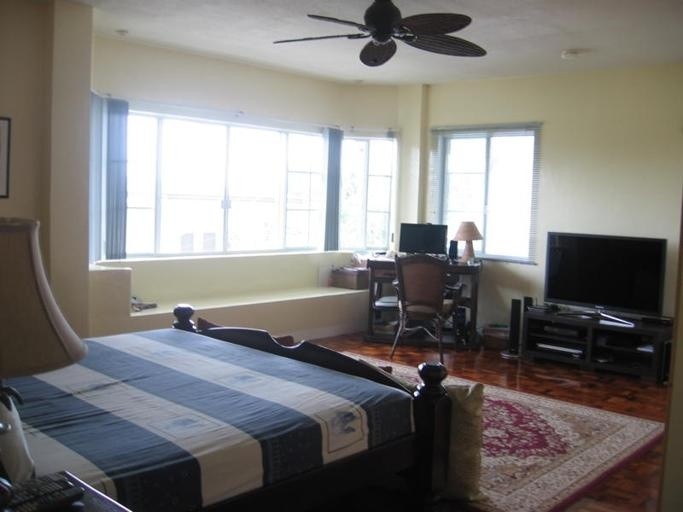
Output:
[388,253,463,366]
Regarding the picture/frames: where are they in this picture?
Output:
[0,116,12,200]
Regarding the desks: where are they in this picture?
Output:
[366,257,484,351]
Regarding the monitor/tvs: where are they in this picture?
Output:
[398,222,448,256]
[543,230,667,326]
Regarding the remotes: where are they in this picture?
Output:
[5,478,75,508]
[13,485,85,512]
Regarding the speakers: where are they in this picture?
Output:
[448,240,458,260]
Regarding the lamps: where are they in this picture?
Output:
[0,217,88,484]
[451,222,483,264]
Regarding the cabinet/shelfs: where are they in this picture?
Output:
[522,305,674,387]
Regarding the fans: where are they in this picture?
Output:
[273,1,487,68]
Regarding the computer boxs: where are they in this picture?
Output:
[454,307,466,343]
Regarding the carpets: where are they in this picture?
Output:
[334,350,665,511]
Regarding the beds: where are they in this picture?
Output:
[0,303,452,511]
